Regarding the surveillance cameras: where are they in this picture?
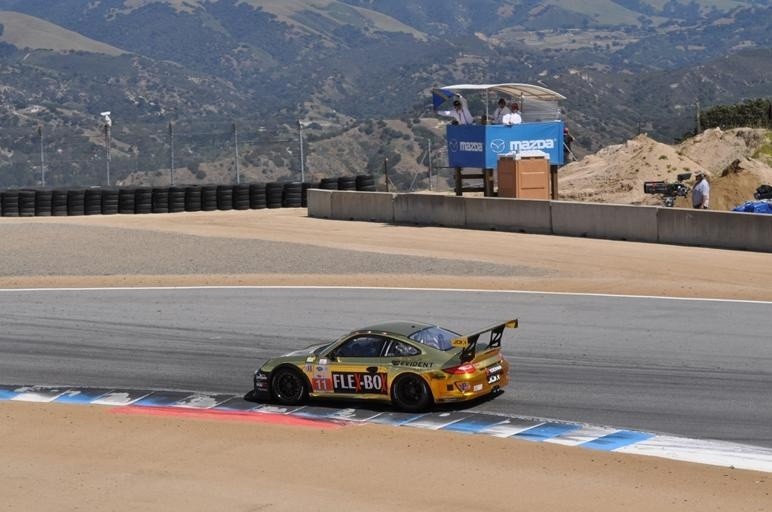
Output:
[100,111,112,124]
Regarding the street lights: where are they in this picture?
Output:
[674,101,701,134]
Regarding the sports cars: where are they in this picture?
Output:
[252,316,523,413]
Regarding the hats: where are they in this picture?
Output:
[693,169,704,176]
[453,101,462,106]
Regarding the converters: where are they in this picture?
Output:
[754,191,772,199]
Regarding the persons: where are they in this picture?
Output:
[563,127,575,165]
[492,98,509,124]
[689,170,708,209]
[433,92,476,125]
[501,102,522,125]
